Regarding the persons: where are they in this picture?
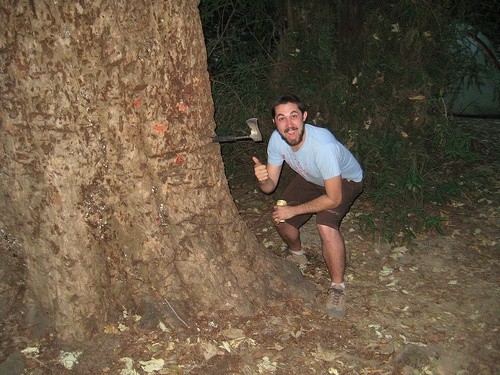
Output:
[251,94,363,316]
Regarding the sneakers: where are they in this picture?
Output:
[283,253,308,268]
[324,283,347,319]
